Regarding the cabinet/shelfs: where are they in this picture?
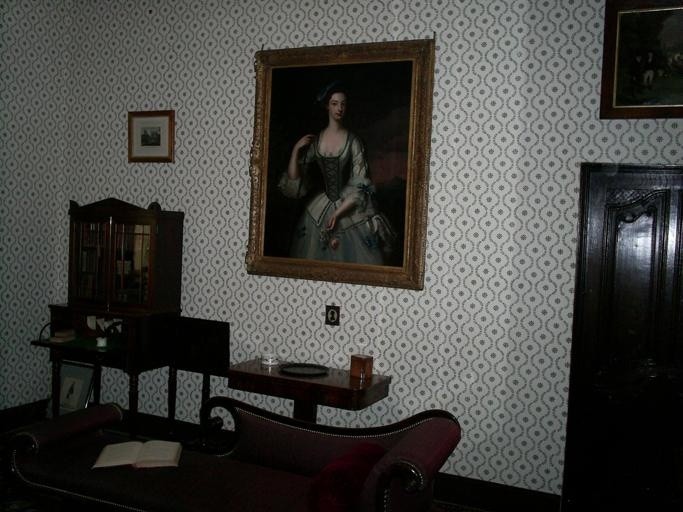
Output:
[30,193,186,421]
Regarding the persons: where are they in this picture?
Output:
[632,53,641,95]
[642,51,654,89]
[65,381,75,399]
[276,81,400,267]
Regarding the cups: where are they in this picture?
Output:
[96,336,107,347]
[262,354,279,365]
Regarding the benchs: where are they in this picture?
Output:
[0,395,465,511]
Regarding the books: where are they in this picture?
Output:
[73,221,150,307]
[90,439,181,470]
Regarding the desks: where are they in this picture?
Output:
[226,351,395,430]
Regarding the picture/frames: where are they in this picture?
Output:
[243,35,437,298]
[125,108,176,165]
[598,0,683,122]
